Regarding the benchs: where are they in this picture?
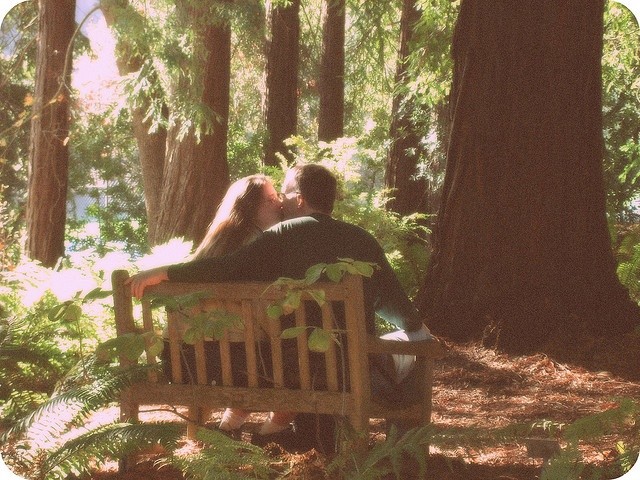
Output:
[112,270,432,473]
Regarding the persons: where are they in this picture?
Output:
[124,164,435,470]
[162,175,301,446]
